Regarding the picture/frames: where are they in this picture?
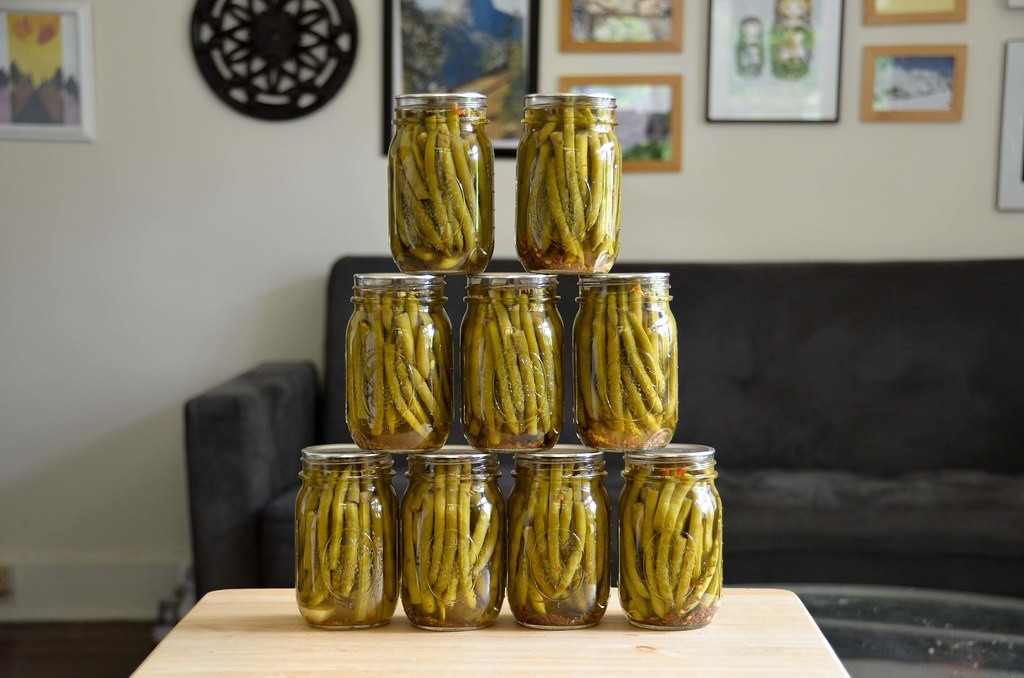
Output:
[384,0,541,158]
[705,0,844,123]
[862,45,967,120]
[863,0,968,26]
[1,0,97,143]
[560,76,682,175]
[559,0,683,54]
[996,39,1024,211]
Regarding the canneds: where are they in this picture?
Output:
[294,443,400,629]
[505,443,612,631]
[387,92,495,276]
[401,445,508,632]
[617,443,723,631]
[572,271,679,454]
[514,94,621,276]
[346,272,453,454]
[457,272,564,453]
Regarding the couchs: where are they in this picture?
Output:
[182,256,1023,672]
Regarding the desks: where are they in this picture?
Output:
[129,586,851,678]
